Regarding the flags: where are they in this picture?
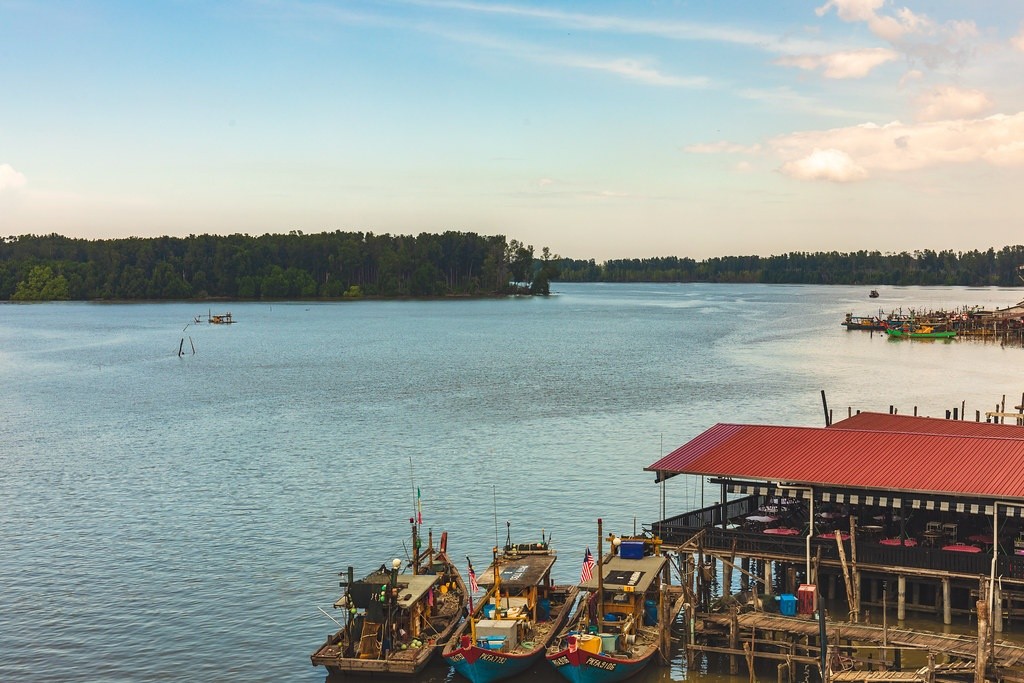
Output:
[469,560,479,591]
[581,548,597,583]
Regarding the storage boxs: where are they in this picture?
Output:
[483,604,495,618]
[780,594,796,616]
[507,608,520,617]
[620,541,645,559]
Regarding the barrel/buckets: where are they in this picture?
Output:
[602,635,615,651]
[644,600,657,627]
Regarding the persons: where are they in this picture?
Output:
[586,591,598,626]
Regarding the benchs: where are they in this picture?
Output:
[490,596,528,609]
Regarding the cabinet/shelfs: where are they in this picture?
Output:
[475,620,518,650]
[926,521,957,545]
[798,584,819,614]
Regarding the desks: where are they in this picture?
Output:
[492,612,529,640]
[817,533,851,541]
[820,512,847,518]
[879,539,918,547]
[745,516,773,523]
[943,545,983,553]
[770,498,800,505]
[924,534,942,549]
[862,526,883,538]
[714,524,740,530]
[967,534,1005,552]
[763,529,800,536]
[759,505,788,513]
[872,515,901,522]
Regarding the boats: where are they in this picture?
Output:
[310,454,470,683]
[543,515,685,683]
[442,486,582,683]
[207,308,237,324]
[840,304,990,339]
